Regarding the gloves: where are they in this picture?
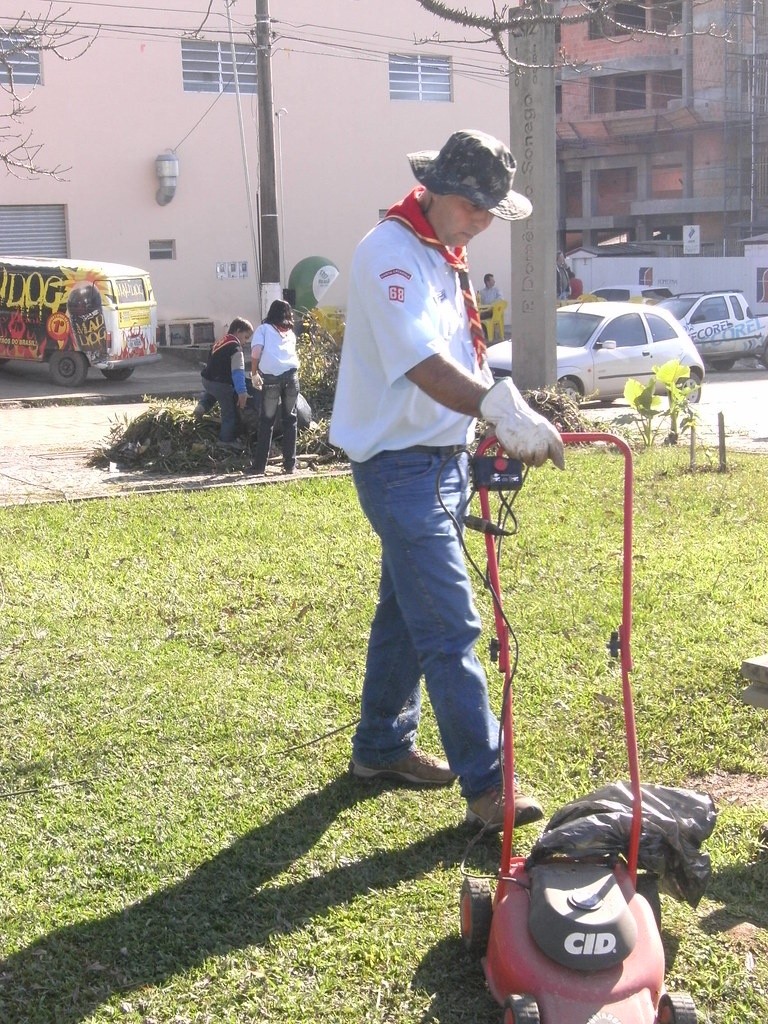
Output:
[478,377,565,471]
[250,371,263,390]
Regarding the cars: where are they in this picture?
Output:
[584,284,675,312]
[482,301,706,412]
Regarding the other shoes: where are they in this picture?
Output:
[286,468,296,474]
[244,466,264,477]
[194,407,205,423]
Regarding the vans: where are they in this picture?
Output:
[0,256,163,388]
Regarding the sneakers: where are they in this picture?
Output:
[466,787,544,833]
[353,745,456,783]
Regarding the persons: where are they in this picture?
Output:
[479,274,504,320]
[327,127,566,836]
[556,250,584,300]
[242,299,301,475]
[193,316,254,443]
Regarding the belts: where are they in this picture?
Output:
[384,445,465,453]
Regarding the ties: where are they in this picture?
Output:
[374,186,486,371]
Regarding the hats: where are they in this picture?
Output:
[407,129,533,221]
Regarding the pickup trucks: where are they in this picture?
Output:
[652,287,768,372]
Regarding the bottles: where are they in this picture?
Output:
[476,290,481,306]
[68,272,107,364]
